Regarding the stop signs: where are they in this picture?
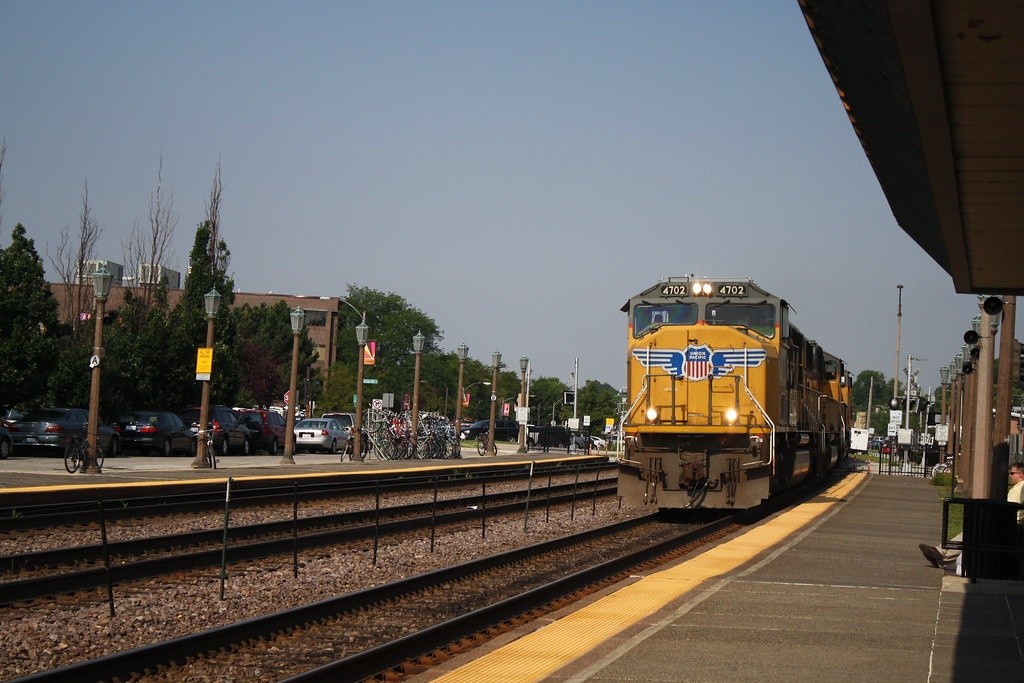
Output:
[284,391,289,406]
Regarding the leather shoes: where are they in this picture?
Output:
[920,548,939,567]
[920,543,945,567]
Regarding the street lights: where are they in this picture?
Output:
[351,319,369,462]
[452,341,470,459]
[84,264,114,473]
[948,292,1000,499]
[484,351,502,457]
[409,328,426,459]
[518,354,529,453]
[191,287,223,467]
[939,363,950,465]
[280,306,307,464]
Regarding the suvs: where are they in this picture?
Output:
[527,426,570,447]
[461,419,519,443]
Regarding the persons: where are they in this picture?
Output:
[919,462,1024,568]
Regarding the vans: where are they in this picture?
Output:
[318,412,356,434]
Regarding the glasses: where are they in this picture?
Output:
[1010,472,1020,476]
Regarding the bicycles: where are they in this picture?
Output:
[476,428,497,456]
[192,421,220,470]
[64,422,105,474]
[340,403,458,462]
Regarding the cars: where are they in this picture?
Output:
[575,435,605,450]
[7,407,120,458]
[177,404,251,455]
[109,407,197,457]
[293,418,350,454]
[867,435,899,455]
[0,426,15,460]
[236,409,297,456]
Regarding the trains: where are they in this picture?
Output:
[614,273,851,524]
